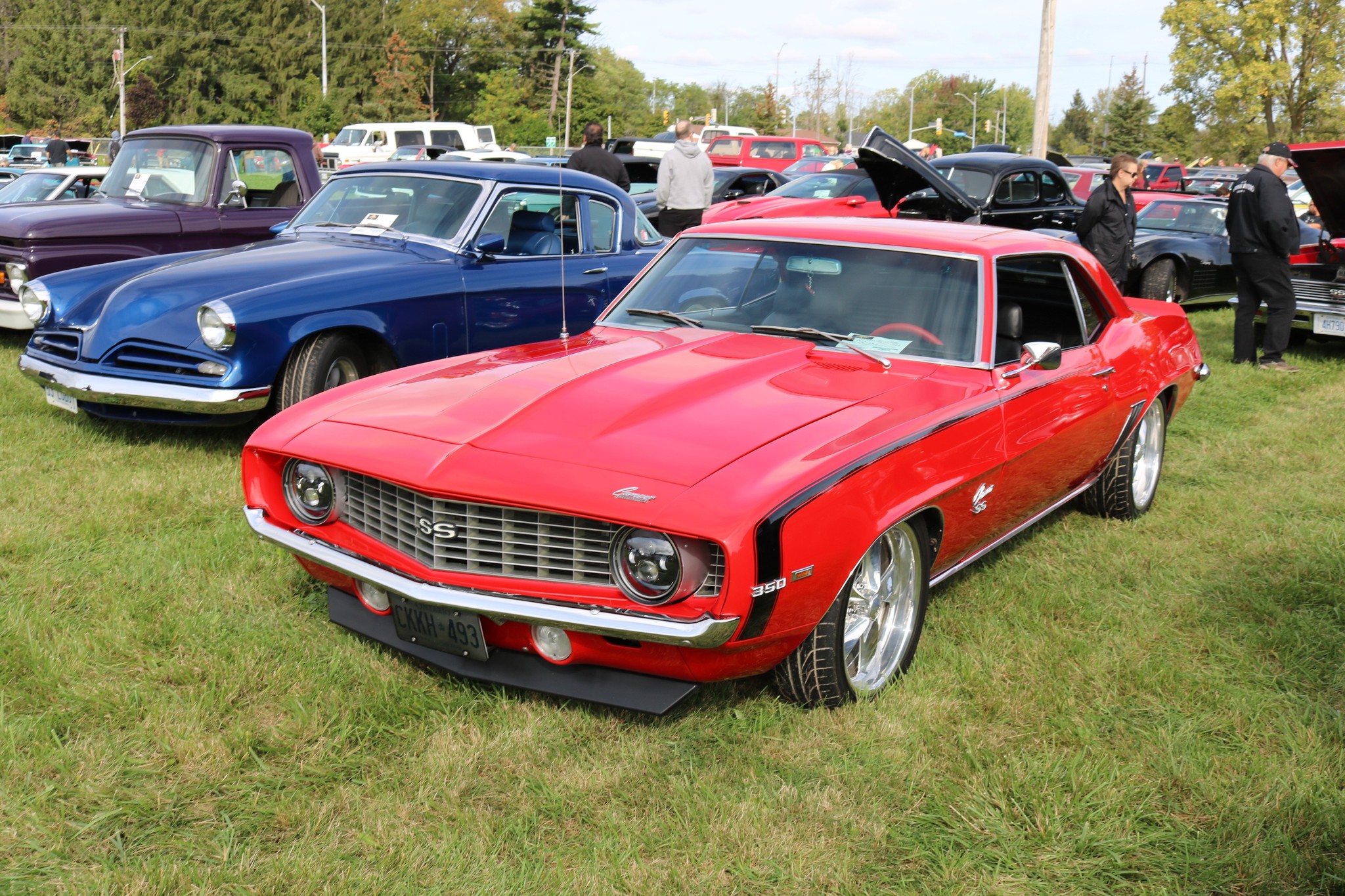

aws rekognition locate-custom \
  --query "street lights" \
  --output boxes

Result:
[953,93,977,149]
[776,42,786,130]
[908,76,927,140]
[564,65,595,148]
[121,56,152,144]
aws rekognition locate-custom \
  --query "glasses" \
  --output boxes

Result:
[1123,170,1137,178]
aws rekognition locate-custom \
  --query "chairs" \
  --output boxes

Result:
[755,277,819,332]
[403,197,452,238]
[496,208,563,258]
[264,181,300,206]
[959,301,1023,362]
[737,181,763,195]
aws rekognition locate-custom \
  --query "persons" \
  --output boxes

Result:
[0,159,10,168]
[308,131,324,167]
[561,121,631,224]
[656,120,715,239]
[231,149,295,183]
[1141,154,1327,234]
[506,142,516,152]
[1077,154,1138,296]
[22,130,73,167]
[1225,141,1303,372]
[108,131,168,168]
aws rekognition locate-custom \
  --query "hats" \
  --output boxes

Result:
[1239,161,1244,164]
[1262,142,1299,168]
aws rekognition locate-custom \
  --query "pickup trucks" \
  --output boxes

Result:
[0,125,455,329]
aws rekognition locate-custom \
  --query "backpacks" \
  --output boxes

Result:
[110,139,121,161]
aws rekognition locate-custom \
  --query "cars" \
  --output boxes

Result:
[18,160,778,429]
[353,123,1345,338]
[241,216,1215,717]
[1,134,238,206]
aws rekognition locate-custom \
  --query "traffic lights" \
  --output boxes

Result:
[705,114,710,127]
[663,110,668,125]
[984,120,992,133]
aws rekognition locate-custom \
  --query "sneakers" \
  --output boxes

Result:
[1260,361,1300,373]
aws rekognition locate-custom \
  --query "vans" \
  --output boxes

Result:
[317,122,495,171]
